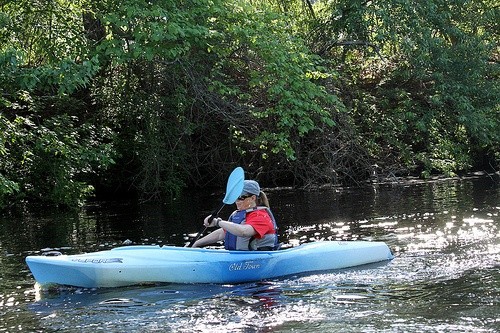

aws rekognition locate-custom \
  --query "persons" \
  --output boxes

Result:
[183,179,278,251]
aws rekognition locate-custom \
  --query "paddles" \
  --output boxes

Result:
[188,166,245,247]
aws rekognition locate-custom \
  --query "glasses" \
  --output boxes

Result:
[237,193,255,201]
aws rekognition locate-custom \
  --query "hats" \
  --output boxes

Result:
[241,179,260,196]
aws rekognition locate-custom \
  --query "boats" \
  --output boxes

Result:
[24,242,392,289]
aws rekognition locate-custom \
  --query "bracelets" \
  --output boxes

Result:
[216,217,222,228]
